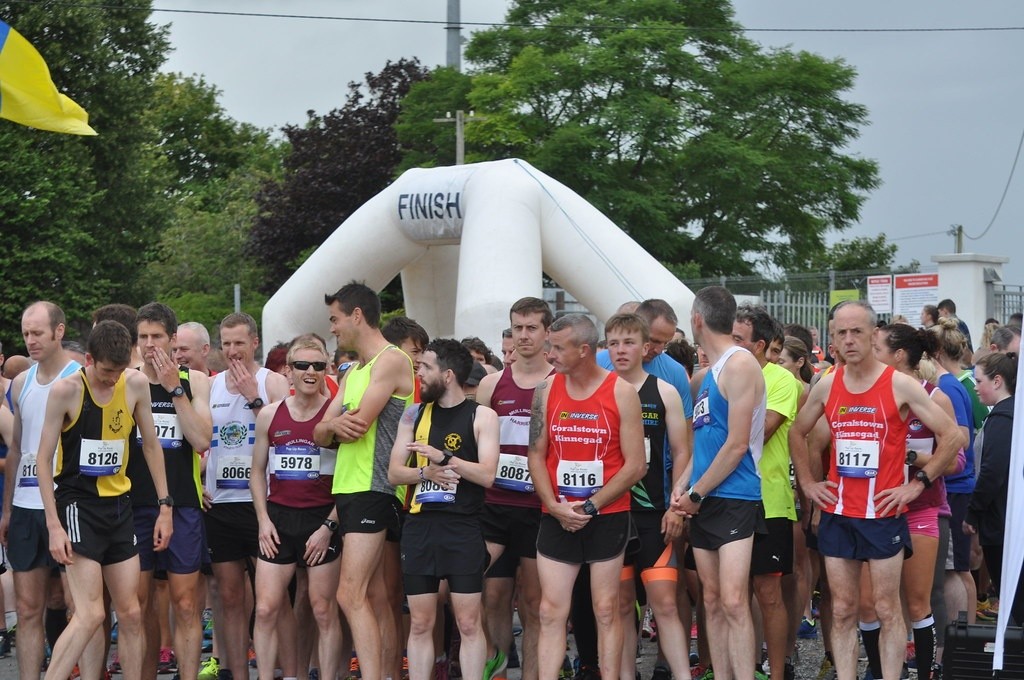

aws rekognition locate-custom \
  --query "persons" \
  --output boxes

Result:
[0,279,1024,680]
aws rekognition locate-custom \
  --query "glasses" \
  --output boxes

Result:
[290,361,327,371]
[337,361,359,372]
[648,342,672,351]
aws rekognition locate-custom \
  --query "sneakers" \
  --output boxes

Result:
[1,607,1023,680]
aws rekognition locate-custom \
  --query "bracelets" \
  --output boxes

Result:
[419,465,426,483]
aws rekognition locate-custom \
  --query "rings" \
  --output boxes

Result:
[317,553,321,557]
[159,364,163,367]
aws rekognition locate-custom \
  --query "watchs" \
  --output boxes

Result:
[437,449,453,466]
[905,450,916,465]
[158,495,173,506]
[913,470,931,489]
[688,486,705,503]
[322,519,338,533]
[169,385,185,398]
[249,398,264,409]
[581,499,598,517]
